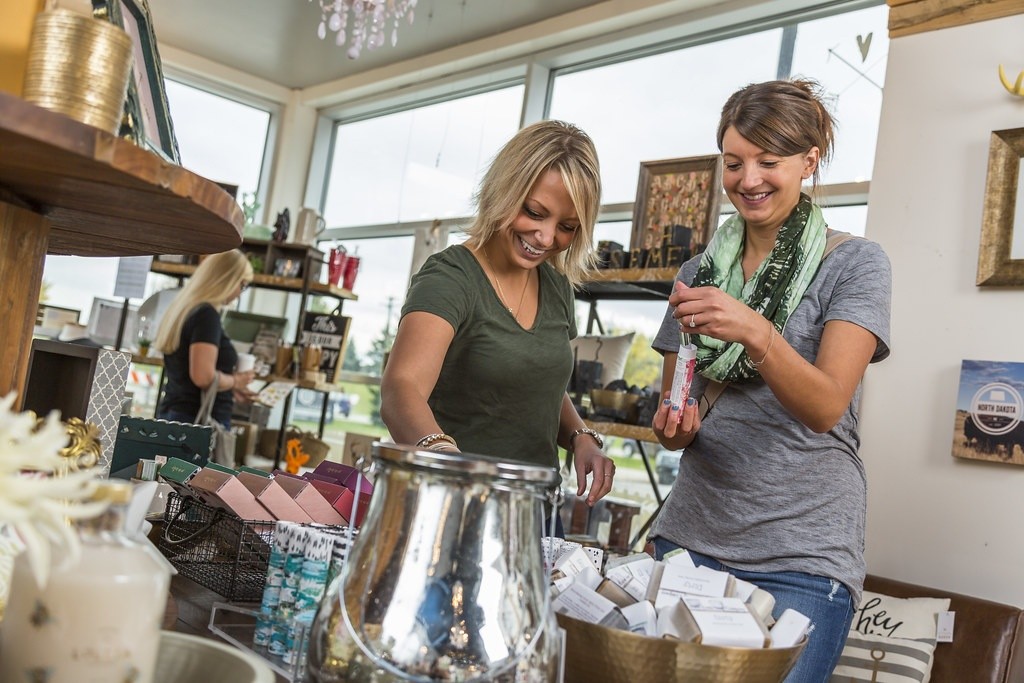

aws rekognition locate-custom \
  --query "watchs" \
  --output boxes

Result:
[569,428,604,452]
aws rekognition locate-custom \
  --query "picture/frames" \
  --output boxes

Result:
[94,0,179,164]
[632,154,725,272]
[975,126,1023,288]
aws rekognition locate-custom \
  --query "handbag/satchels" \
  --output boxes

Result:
[195,370,236,469]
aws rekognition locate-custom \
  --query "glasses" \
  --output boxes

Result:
[239,281,248,292]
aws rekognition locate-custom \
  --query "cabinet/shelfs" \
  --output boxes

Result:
[150,256,361,445]
[384,248,738,443]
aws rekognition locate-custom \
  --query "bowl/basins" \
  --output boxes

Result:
[590,389,640,420]
[153,631,275,683]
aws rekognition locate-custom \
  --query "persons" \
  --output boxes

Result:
[642,80,892,683]
[414,434,457,451]
[377,122,616,540]
[153,248,262,461]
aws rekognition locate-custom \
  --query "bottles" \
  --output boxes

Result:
[0,479,173,683]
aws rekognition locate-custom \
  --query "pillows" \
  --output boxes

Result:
[832,590,951,683]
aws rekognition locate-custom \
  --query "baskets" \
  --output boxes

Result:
[159,490,277,602]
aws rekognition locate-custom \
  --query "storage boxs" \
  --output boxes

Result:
[157,458,375,599]
[19,339,132,479]
[554,547,814,651]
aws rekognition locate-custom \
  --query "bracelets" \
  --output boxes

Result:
[748,320,774,367]
[232,376,236,389]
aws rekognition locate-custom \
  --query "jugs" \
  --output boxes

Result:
[293,208,326,245]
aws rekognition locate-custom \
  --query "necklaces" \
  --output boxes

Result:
[481,243,531,320]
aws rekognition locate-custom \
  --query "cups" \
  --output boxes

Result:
[325,248,348,287]
[276,346,293,377]
[341,257,359,292]
[237,353,257,373]
[302,343,323,371]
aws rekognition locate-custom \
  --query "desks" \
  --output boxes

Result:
[0,98,247,412]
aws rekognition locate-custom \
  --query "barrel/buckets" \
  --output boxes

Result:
[306,441,570,682]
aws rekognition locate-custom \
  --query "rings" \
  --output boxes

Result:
[604,473,612,477]
[690,314,696,328]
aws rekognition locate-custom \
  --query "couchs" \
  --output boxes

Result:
[832,573,1024,683]
